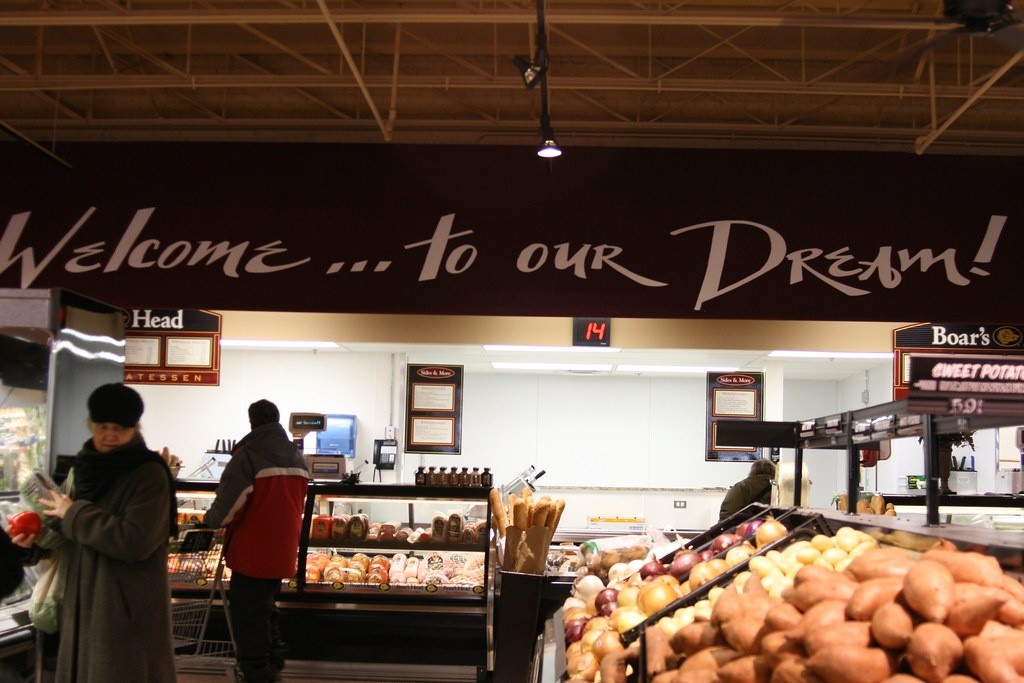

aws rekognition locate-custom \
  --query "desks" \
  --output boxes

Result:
[493,502,1024,683]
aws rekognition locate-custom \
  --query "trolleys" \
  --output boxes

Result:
[163,526,246,683]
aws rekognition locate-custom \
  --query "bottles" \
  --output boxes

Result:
[447,467,459,487]
[481,468,493,488]
[470,467,481,488]
[415,466,426,485]
[459,467,470,487]
[437,467,448,486]
[426,467,437,486]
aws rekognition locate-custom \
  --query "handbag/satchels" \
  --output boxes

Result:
[28,548,64,635]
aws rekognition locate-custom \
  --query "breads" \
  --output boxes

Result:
[365,513,488,547]
[306,552,391,583]
[488,486,566,540]
[838,492,895,515]
[160,447,181,467]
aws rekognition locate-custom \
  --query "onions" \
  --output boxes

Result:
[564,516,788,657]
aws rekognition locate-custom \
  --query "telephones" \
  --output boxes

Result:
[372,439,397,470]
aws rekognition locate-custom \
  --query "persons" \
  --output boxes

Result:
[202,399,310,683]
[720,459,776,521]
[5,382,177,683]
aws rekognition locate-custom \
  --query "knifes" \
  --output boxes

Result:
[950,455,975,472]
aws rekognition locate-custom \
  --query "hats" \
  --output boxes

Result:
[87,382,144,426]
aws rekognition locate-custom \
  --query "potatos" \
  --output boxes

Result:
[563,527,1024,683]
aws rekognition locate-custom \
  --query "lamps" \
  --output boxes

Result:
[511,55,544,86]
[537,115,563,158]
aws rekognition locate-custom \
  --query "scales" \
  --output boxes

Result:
[289,412,347,480]
